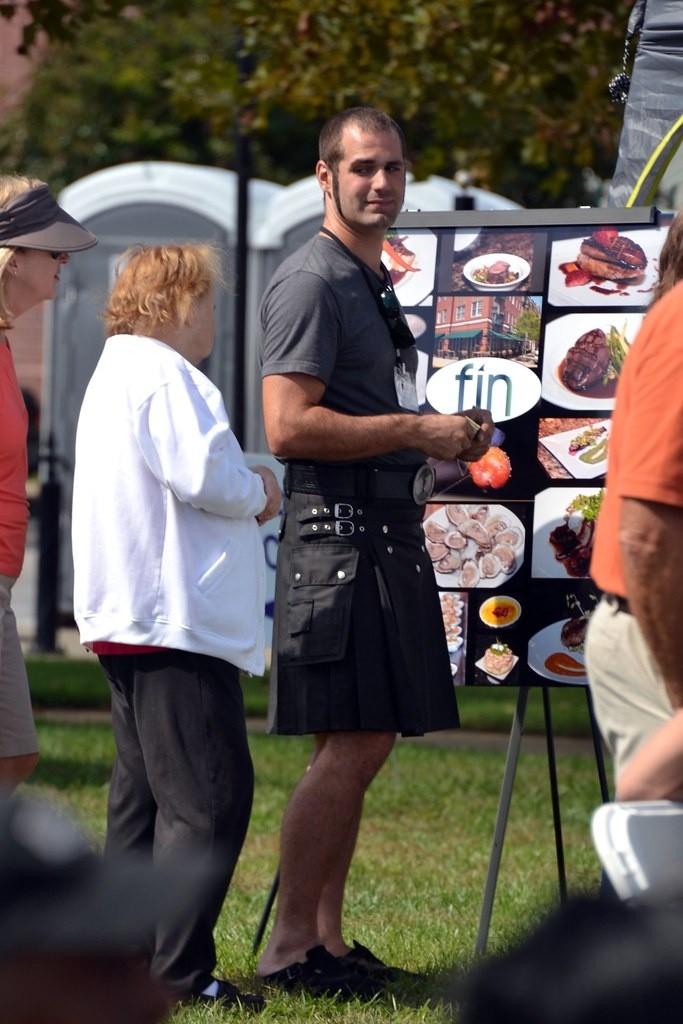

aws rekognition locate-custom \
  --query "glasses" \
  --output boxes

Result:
[18,246,59,260]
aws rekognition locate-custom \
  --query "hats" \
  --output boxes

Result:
[0,184,98,252]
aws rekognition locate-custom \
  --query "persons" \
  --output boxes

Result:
[0,175,99,803]
[457,894,683,1024]
[583,209,683,801]
[0,791,220,1024]
[71,243,283,1019]
[254,107,495,1004]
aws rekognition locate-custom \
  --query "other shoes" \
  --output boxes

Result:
[174,977,264,1014]
[263,943,386,1001]
[340,940,426,995]
